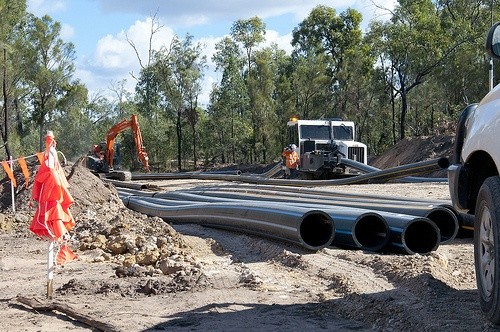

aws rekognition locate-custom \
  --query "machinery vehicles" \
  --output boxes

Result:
[286,117,368,180]
[78,113,151,182]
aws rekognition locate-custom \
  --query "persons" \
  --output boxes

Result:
[282,144,300,179]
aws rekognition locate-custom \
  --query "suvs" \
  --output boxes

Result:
[445,20,500,326]
